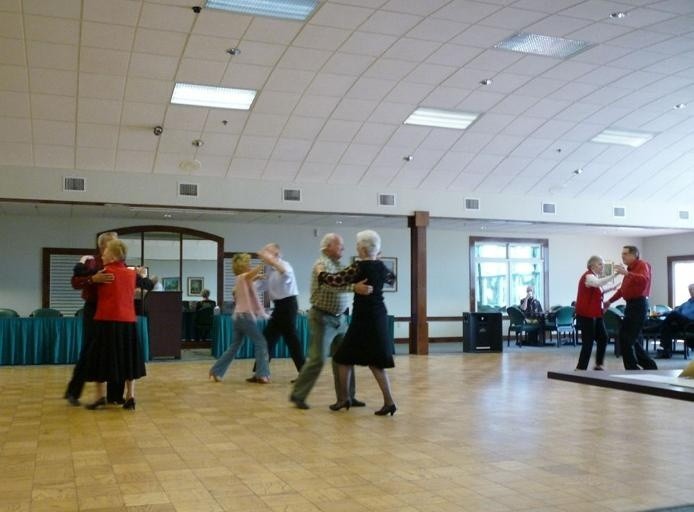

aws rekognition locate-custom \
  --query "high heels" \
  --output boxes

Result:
[329,399,351,411]
[374,404,396,416]
[208,370,223,381]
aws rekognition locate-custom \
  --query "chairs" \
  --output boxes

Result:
[76,307,86,315]
[266,308,274,315]
[0,307,18,317]
[29,308,62,316]
[477,302,694,359]
[194,300,216,332]
[222,300,235,315]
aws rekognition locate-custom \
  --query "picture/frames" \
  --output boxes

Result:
[187,277,204,296]
[162,277,180,291]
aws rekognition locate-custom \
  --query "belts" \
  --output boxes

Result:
[312,306,341,318]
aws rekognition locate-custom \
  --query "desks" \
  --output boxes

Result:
[1,315,150,365]
[546,369,694,403]
[182,311,216,342]
[210,311,396,360]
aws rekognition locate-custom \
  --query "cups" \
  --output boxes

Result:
[650,304,656,316]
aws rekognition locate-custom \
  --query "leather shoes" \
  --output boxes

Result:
[290,394,309,409]
[247,375,272,383]
[352,397,365,407]
[68,395,136,412]
[654,353,671,359]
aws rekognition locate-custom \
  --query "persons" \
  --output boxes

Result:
[602,245,657,371]
[245,243,306,383]
[573,255,623,371]
[63,231,165,411]
[207,253,272,384]
[223,287,238,315]
[657,283,694,358]
[289,233,373,409]
[315,229,397,417]
[520,287,546,343]
[200,289,209,301]
